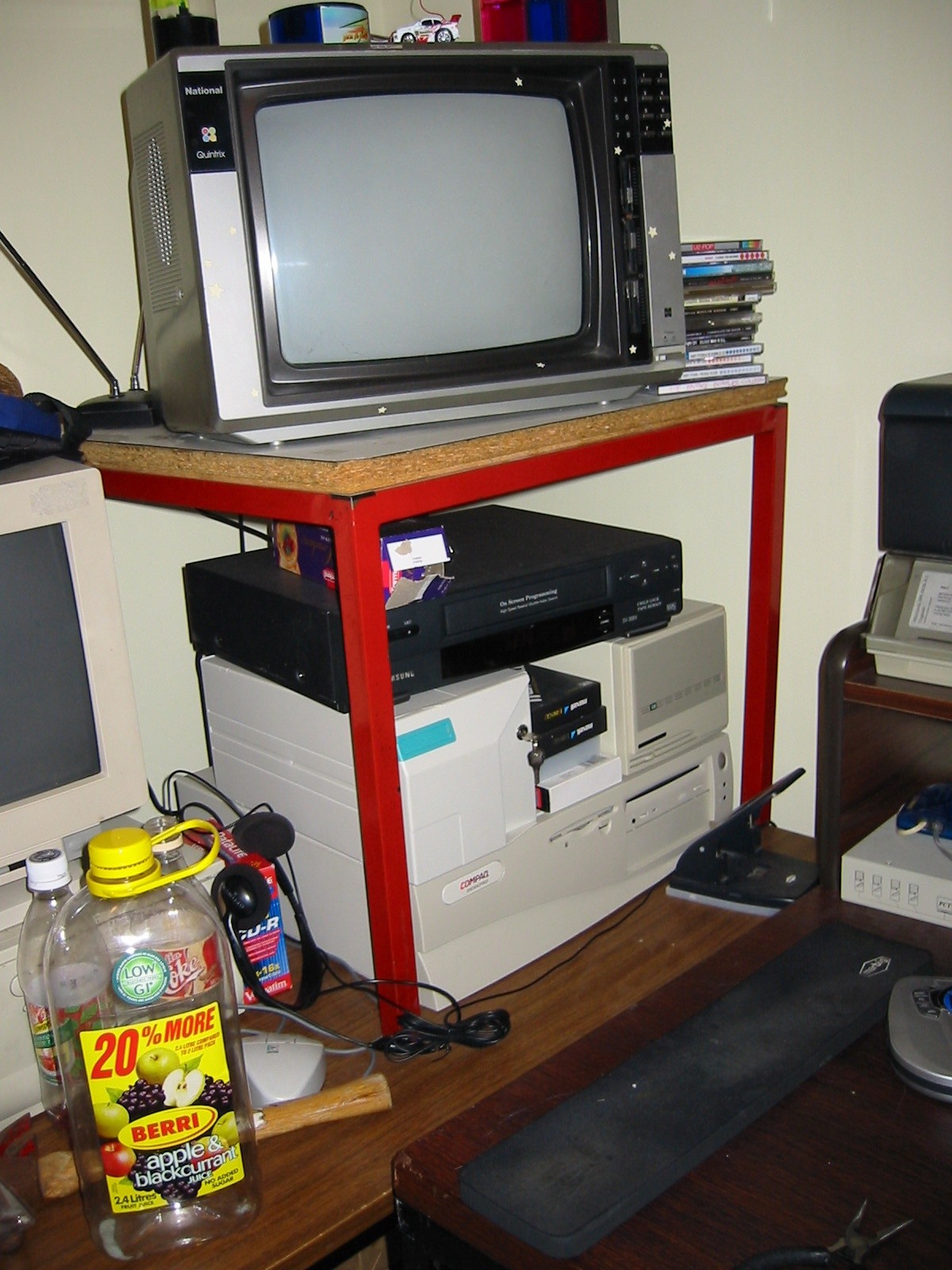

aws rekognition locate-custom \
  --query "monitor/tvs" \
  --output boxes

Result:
[0,453,148,889]
[120,45,690,450]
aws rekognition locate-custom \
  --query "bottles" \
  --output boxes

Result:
[43,818,263,1260]
[137,815,234,1089]
[16,851,121,1126]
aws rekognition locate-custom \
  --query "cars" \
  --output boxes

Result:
[389,14,462,44]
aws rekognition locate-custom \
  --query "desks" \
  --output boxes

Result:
[390,619,952,1270]
[80,375,790,1039]
[0,823,807,1270]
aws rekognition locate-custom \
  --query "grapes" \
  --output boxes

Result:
[116,1076,233,1205]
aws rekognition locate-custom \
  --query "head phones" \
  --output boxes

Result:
[210,811,321,1012]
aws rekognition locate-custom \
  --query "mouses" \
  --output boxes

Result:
[241,1031,327,1105]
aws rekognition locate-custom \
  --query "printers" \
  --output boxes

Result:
[856,369,952,689]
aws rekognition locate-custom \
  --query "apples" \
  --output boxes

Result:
[191,1111,240,1173]
[92,1101,136,1178]
[136,1047,206,1108]
